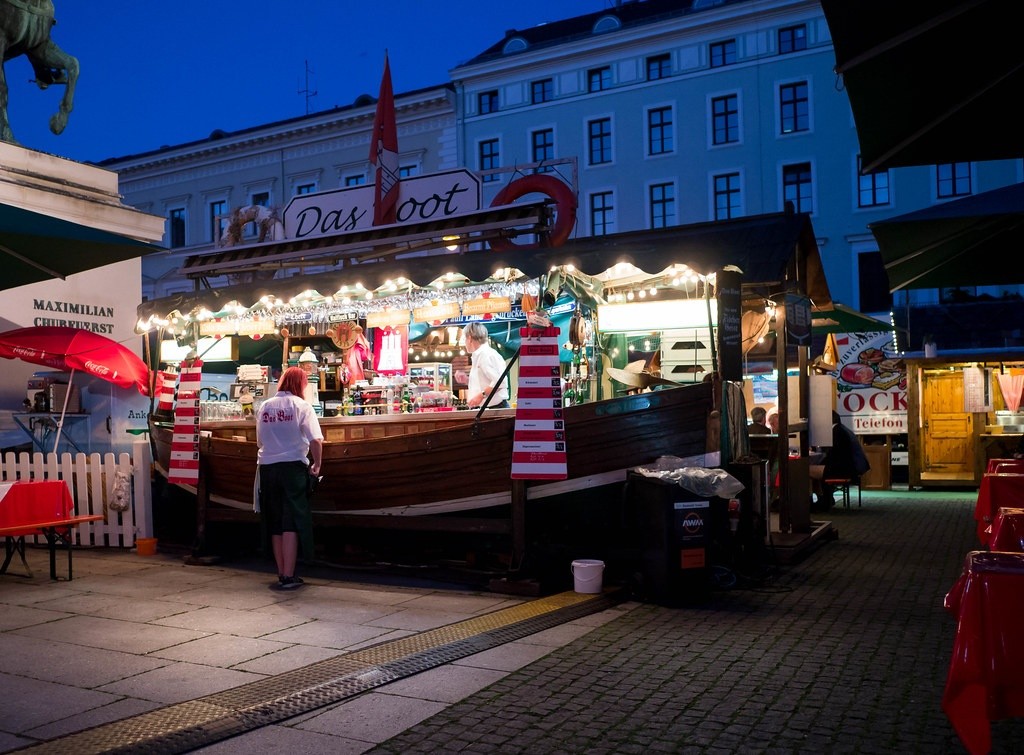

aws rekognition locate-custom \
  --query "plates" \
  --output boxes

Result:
[50,384,80,412]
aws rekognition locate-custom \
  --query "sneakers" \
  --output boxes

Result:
[278,574,304,587]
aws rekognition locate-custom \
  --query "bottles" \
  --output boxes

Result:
[344,372,476,416]
[200,401,245,420]
[561,347,589,408]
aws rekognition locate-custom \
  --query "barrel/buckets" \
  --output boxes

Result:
[136,538,157,555]
[571,559,605,593]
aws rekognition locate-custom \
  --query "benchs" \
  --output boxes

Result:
[824,476,862,509]
[0,515,105,581]
[946,456,1024,755]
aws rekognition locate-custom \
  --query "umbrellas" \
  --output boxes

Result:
[869,184,1024,295]
[821,0,1024,175]
[0,326,164,454]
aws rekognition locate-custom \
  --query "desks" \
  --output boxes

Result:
[0,478,74,577]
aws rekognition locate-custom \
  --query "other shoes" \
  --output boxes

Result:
[818,497,835,506]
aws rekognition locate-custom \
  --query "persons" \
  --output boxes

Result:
[462,323,510,410]
[257,366,324,588]
[748,407,871,512]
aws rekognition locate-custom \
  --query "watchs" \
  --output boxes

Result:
[482,391,486,398]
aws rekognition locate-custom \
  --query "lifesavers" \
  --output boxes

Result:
[481,171,577,251]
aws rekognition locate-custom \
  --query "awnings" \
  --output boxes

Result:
[768,302,895,338]
